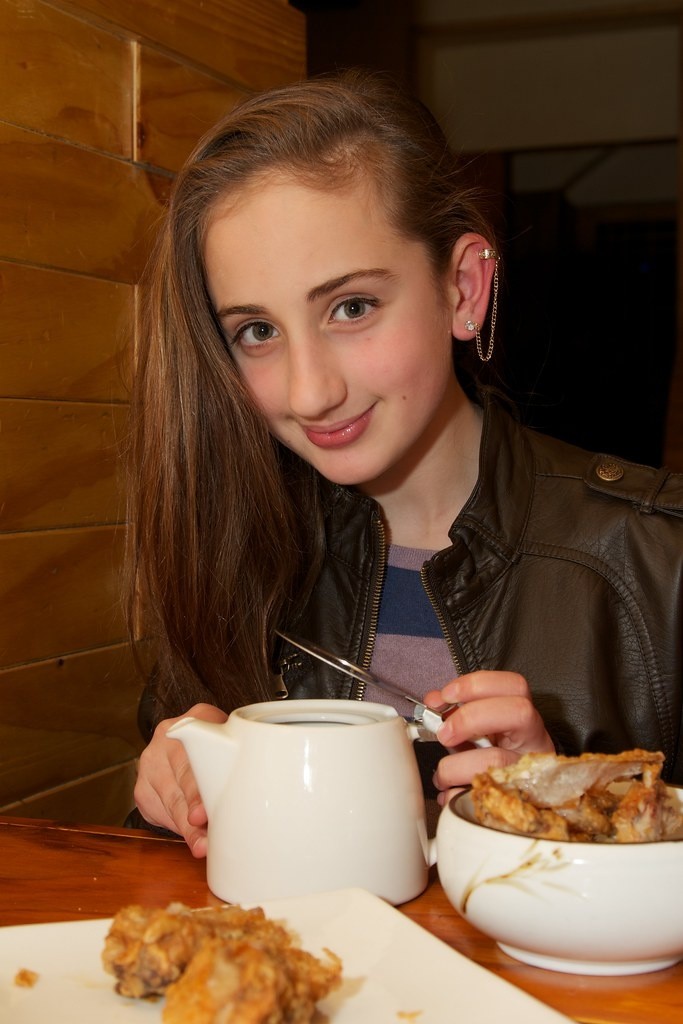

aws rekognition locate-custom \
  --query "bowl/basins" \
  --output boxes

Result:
[437,786,683,975]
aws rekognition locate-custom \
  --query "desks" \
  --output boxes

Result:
[2,807,682,1022]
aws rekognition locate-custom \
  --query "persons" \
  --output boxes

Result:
[108,65,683,859]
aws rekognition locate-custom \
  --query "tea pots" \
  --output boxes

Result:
[163,631,494,907]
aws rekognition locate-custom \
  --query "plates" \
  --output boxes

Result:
[0,886,578,1024]
